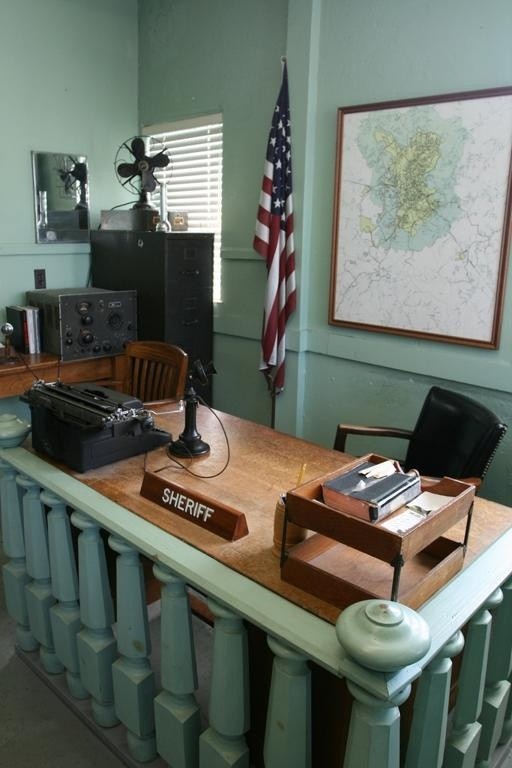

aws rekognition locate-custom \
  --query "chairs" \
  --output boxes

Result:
[97,340,189,407]
[333,387,508,496]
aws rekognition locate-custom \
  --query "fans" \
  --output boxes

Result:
[114,135,175,211]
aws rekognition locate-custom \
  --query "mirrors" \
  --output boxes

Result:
[31,151,91,245]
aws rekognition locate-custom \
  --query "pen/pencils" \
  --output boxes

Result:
[297,462,306,486]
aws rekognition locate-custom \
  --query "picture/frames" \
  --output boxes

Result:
[328,86,512,350]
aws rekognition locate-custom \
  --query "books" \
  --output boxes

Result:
[319,460,422,524]
[5,304,44,354]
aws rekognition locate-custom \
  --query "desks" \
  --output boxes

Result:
[18,396,511,624]
[0,342,134,398]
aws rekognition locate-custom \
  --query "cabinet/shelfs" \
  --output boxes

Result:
[89,229,215,409]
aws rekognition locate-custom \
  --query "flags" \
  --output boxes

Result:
[250,55,295,391]
